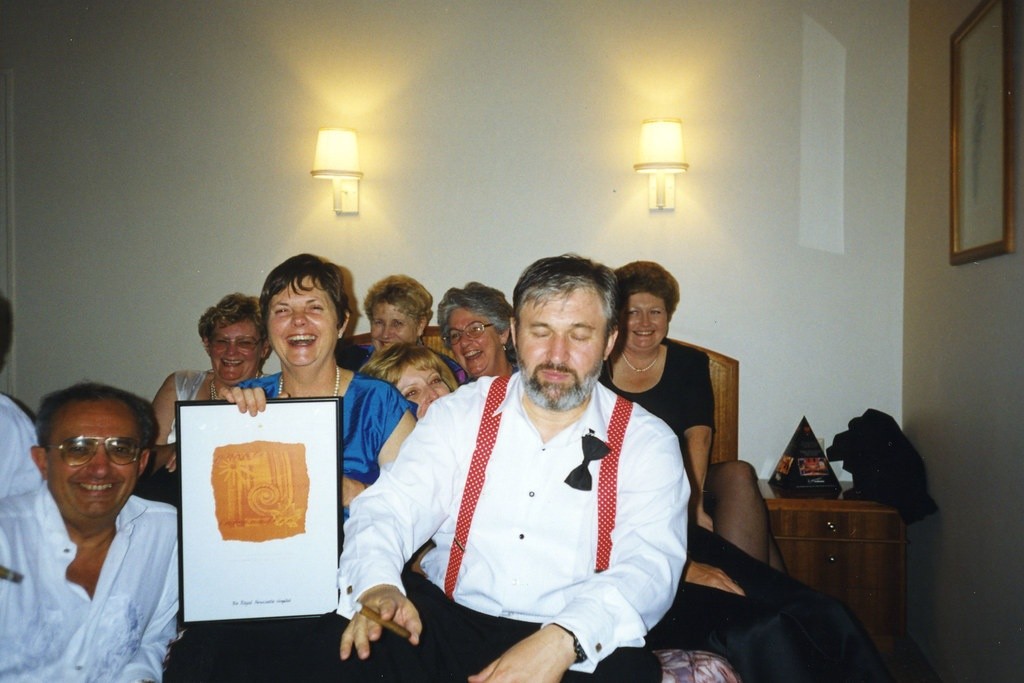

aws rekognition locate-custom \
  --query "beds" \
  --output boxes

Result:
[352,327,740,683]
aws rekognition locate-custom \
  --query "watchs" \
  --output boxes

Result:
[551,623,587,664]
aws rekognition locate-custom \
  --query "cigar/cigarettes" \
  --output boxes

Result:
[353,601,412,641]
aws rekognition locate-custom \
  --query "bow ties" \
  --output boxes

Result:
[563,431,611,491]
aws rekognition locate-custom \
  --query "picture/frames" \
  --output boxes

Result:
[174,396,344,628]
[949,0,1016,266]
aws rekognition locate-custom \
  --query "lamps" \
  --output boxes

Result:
[632,116,688,213]
[309,125,364,214]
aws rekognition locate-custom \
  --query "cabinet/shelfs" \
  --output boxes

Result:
[757,479,909,654]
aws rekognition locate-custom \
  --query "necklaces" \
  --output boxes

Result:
[622,345,660,372]
[210,371,264,400]
[278,365,340,397]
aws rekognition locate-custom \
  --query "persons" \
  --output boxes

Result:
[0,293,40,498]
[359,274,519,382]
[0,382,178,683]
[355,342,896,682]
[216,253,419,523]
[598,261,787,574]
[334,254,692,683]
[152,292,273,473]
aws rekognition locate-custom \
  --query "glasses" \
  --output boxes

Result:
[447,319,495,345]
[44,434,151,466]
[207,330,265,353]
